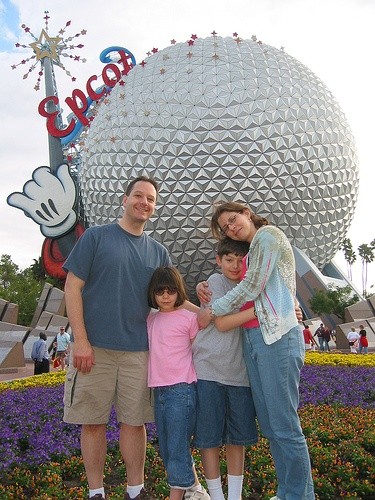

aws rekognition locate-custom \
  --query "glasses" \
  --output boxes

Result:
[219,210,240,236]
[154,288,178,296]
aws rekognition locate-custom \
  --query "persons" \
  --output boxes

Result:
[196,202,315,500]
[31,326,71,375]
[303,323,368,354]
[146,266,210,500]
[191,237,303,500]
[62,177,201,500]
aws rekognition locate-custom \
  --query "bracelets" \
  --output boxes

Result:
[254,310,257,317]
[209,305,215,321]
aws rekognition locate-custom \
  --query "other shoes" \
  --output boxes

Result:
[184,486,210,500]
[123,488,159,500]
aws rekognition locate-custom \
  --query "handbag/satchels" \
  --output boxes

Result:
[349,342,354,346]
